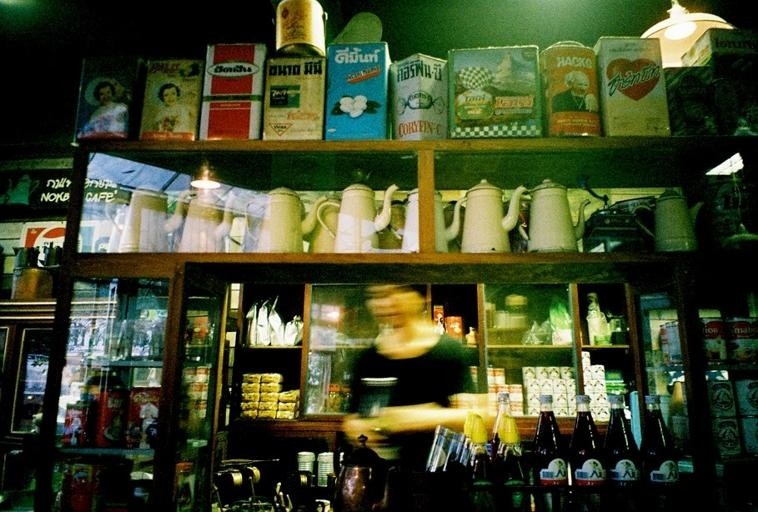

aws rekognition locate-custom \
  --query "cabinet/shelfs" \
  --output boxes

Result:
[226,280,644,472]
[34,137,758,512]
[0,294,212,510]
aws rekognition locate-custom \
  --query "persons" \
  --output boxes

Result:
[341,266,481,468]
[89,81,127,131]
[153,82,196,135]
[552,69,598,113]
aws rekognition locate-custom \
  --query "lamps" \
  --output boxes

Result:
[641,0,732,65]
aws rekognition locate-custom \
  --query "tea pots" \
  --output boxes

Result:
[390,185,468,252]
[307,194,340,256]
[332,434,399,509]
[376,194,410,250]
[106,185,190,252]
[512,178,591,252]
[445,179,528,254]
[243,186,327,252]
[175,189,238,252]
[316,183,398,254]
[632,186,705,251]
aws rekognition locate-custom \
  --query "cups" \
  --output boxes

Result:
[425,423,466,474]
[318,452,336,487]
[297,451,314,473]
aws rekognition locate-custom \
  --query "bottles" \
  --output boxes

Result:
[604,394,640,483]
[665,321,682,359]
[491,392,512,452]
[570,395,606,487]
[668,381,686,439]
[463,411,491,481]
[700,317,727,362]
[640,395,680,483]
[532,395,568,487]
[706,380,736,418]
[713,417,741,459]
[169,462,195,512]
[741,416,758,453]
[495,412,526,482]
[735,380,758,417]
[658,324,669,354]
[727,318,758,365]
